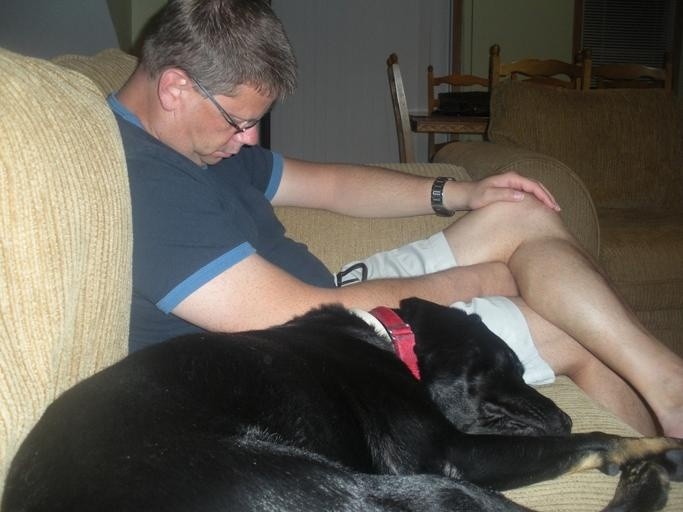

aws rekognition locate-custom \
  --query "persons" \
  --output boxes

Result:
[101,1,682,438]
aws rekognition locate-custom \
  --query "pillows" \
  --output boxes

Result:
[489,77,682,215]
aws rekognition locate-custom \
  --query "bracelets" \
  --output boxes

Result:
[431,175,458,217]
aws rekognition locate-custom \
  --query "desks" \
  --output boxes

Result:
[409,115,490,134]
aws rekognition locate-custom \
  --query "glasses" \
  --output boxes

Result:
[190,77,257,133]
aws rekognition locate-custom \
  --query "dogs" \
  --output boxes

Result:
[0,295,681,511]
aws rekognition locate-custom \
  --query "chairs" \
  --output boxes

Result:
[489,44,591,94]
[428,64,488,162]
[575,51,672,92]
[387,51,418,163]
[431,77,683,359]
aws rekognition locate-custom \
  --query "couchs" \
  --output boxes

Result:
[1,47,648,511]
